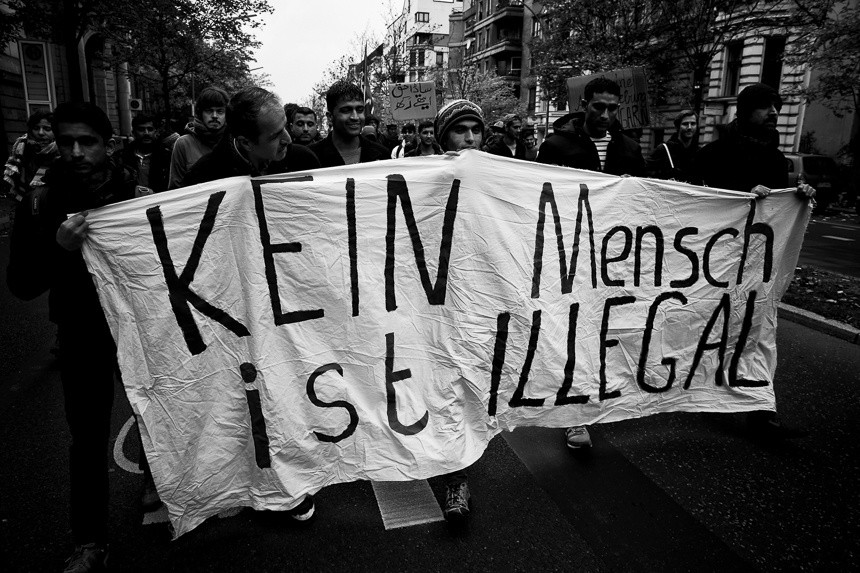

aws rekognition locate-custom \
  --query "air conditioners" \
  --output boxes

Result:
[129,99,142,111]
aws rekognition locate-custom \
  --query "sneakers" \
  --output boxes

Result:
[142,470,161,511]
[444,479,470,518]
[566,424,593,449]
[61,544,107,573]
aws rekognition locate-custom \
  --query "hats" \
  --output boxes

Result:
[737,84,781,118]
[362,126,378,142]
[434,99,485,146]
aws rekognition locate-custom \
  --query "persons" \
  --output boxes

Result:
[691,84,816,430]
[4,74,707,573]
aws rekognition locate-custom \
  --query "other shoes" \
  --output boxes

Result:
[292,496,316,521]
